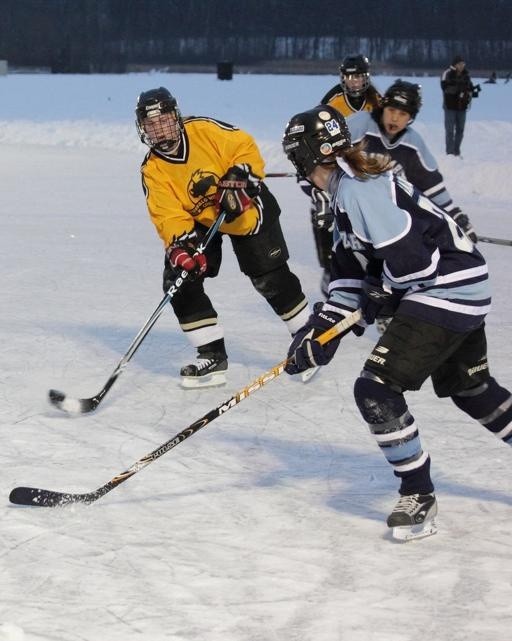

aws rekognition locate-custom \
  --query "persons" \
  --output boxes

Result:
[312,55,385,266]
[314,78,475,296]
[136,89,323,389]
[283,105,512,542]
[442,57,479,156]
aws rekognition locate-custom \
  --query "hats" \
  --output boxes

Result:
[452,54,463,65]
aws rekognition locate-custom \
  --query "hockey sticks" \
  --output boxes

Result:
[9,308,364,505]
[50,209,226,414]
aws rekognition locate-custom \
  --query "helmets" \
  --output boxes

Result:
[378,79,422,119]
[340,54,371,97]
[135,87,182,150]
[281,104,351,183]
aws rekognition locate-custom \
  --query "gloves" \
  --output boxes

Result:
[362,272,388,325]
[285,310,350,376]
[215,168,253,223]
[166,242,207,281]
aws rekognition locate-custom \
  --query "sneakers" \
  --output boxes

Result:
[181,353,227,377]
[387,494,438,527]
